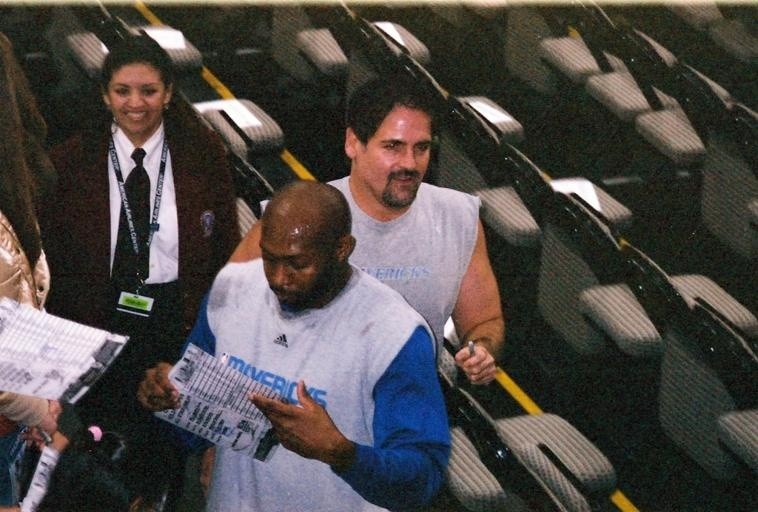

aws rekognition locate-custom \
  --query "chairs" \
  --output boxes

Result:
[25,1,758,512]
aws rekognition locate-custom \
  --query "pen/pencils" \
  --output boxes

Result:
[40,430,52,443]
[468,341,489,387]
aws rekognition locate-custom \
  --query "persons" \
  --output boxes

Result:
[200,75,505,501]
[136,180,452,512]
[40,43,241,512]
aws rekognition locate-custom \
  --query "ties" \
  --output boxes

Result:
[112,148,150,291]
